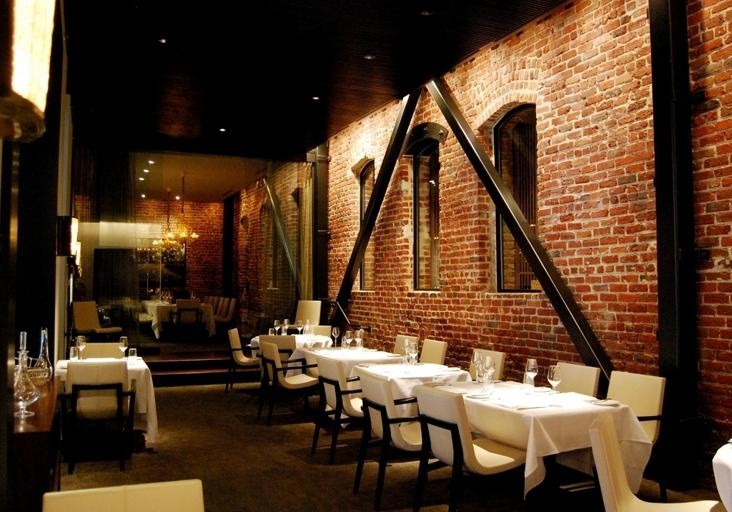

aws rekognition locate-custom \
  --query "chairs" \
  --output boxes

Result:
[42,478,206,512]
[57,363,136,474]
[73,342,127,358]
[72,301,123,335]
[119,294,236,341]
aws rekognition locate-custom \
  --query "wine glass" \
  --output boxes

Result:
[346,331,353,352]
[296,320,303,335]
[355,330,363,353]
[547,365,564,405]
[525,359,538,392]
[484,355,495,391]
[409,343,419,369]
[274,320,281,335]
[332,327,340,350]
[147,288,173,305]
[119,336,128,360]
[70,347,78,361]
[402,339,410,366]
[305,320,317,337]
[284,319,290,335]
[191,292,196,300]
[367,348,377,352]
[77,335,86,360]
[471,350,482,382]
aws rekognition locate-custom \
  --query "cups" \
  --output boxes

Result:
[341,336,347,350]
[129,348,137,365]
[27,368,45,385]
[269,329,273,335]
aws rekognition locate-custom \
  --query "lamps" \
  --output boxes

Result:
[150,173,200,261]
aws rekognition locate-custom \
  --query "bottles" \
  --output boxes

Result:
[35,327,53,382]
[136,246,186,263]
[15,332,40,417]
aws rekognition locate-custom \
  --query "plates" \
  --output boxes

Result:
[442,367,460,371]
[466,393,490,399]
[386,353,401,356]
[360,362,376,367]
[501,381,518,386]
[534,387,549,391]
[592,400,619,406]
[133,356,142,360]
[435,385,468,393]
